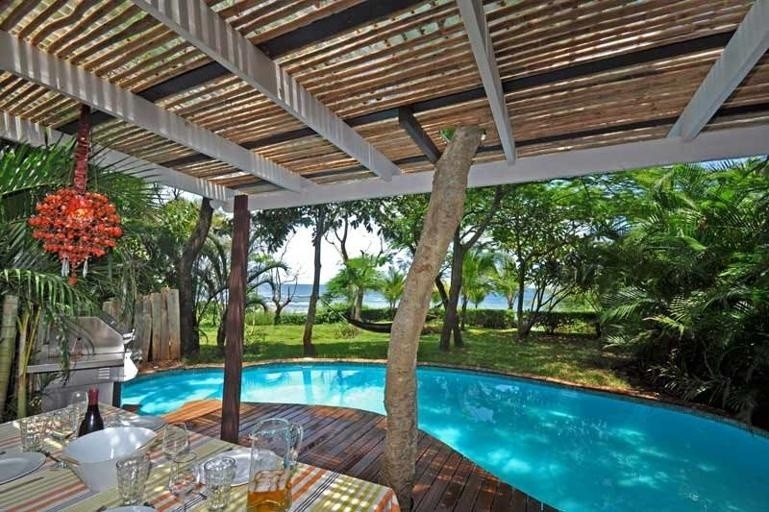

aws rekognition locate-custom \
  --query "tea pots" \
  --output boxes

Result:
[246,417,303,512]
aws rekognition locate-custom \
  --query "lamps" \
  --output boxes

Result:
[28,103,123,282]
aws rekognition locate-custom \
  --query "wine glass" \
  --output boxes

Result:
[162,421,200,512]
[51,409,78,470]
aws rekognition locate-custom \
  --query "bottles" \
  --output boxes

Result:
[78,388,103,437]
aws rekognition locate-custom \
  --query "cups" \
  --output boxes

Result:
[204,456,237,511]
[116,453,151,506]
[19,416,44,452]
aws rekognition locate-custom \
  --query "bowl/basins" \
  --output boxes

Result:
[62,427,158,492]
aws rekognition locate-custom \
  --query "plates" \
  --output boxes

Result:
[1,451,46,485]
[114,415,167,431]
[199,447,250,486]
